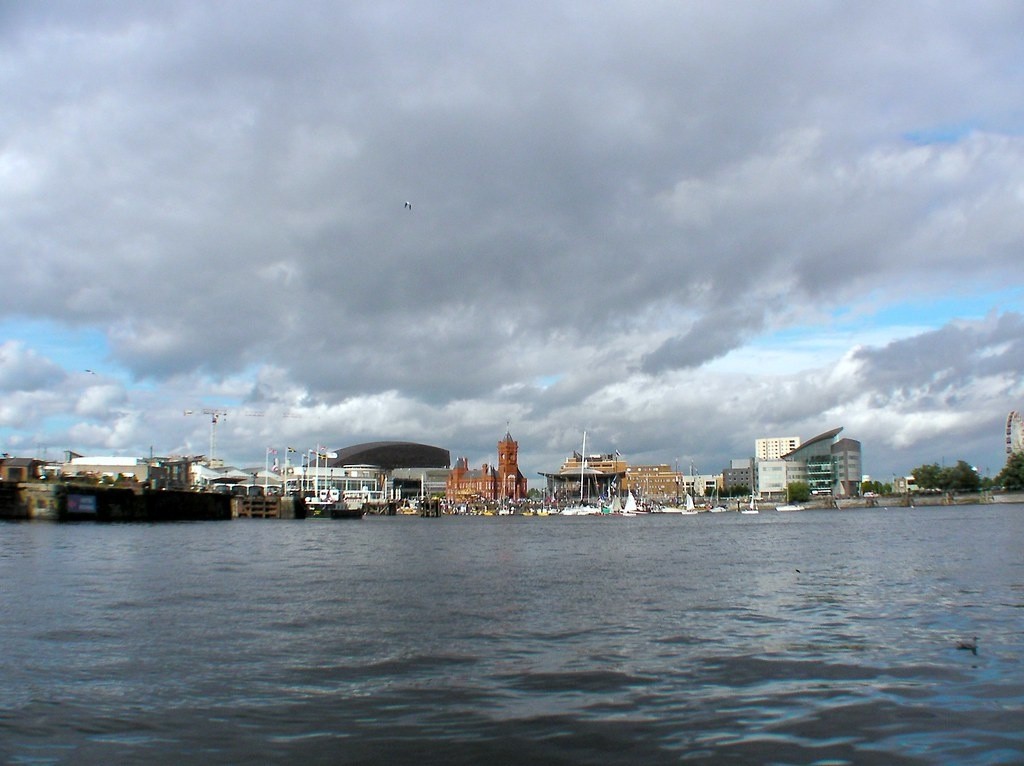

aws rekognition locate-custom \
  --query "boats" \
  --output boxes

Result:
[476,430,763,516]
[774,503,806,512]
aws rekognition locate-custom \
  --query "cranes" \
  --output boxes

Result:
[181,406,306,470]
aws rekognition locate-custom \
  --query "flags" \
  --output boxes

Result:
[610,476,617,493]
[267,445,337,471]
[616,450,621,457]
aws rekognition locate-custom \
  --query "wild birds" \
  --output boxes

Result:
[405,202,411,210]
[796,569,800,573]
[85,370,95,374]
[955,636,980,648]
